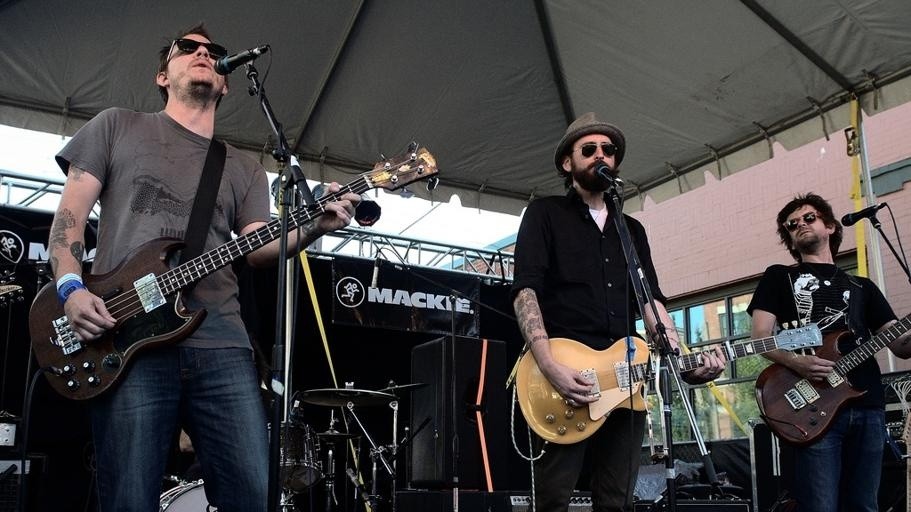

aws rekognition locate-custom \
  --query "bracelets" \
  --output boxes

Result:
[56,273,84,306]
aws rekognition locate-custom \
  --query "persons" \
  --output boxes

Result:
[509,111,728,512]
[745,193,911,512]
[49,22,363,512]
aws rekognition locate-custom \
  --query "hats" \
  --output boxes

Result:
[553,110,624,176]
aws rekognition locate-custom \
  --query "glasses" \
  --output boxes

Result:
[160,37,226,69]
[780,208,823,232]
[567,139,620,159]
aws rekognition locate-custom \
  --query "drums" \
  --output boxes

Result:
[280,419,320,491]
[158,478,211,512]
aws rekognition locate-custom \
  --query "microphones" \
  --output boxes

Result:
[594,162,624,188]
[842,203,884,226]
[214,44,269,74]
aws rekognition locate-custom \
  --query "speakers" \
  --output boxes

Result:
[411,336,507,488]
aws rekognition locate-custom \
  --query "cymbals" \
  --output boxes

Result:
[304,389,399,408]
[378,382,423,389]
[317,430,355,438]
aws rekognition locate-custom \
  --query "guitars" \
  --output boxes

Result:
[756,313,911,443]
[30,141,440,400]
[516,324,823,445]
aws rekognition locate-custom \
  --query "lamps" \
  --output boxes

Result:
[269,171,383,226]
